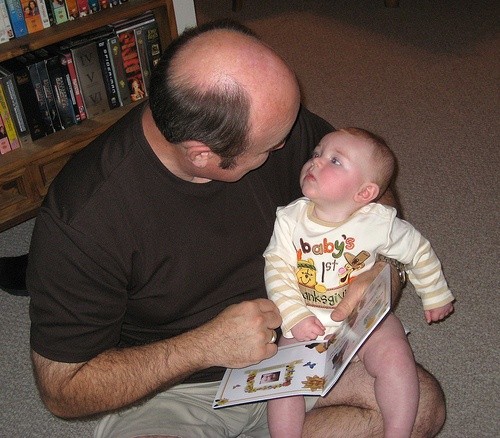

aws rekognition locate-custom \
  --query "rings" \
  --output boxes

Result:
[268,330,276,344]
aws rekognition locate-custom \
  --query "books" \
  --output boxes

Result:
[1,15,169,156]
[1,1,133,44]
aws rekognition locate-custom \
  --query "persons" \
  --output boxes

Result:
[28,19,447,437]
[262,127,457,438]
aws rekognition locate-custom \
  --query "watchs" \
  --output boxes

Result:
[376,253,407,286]
